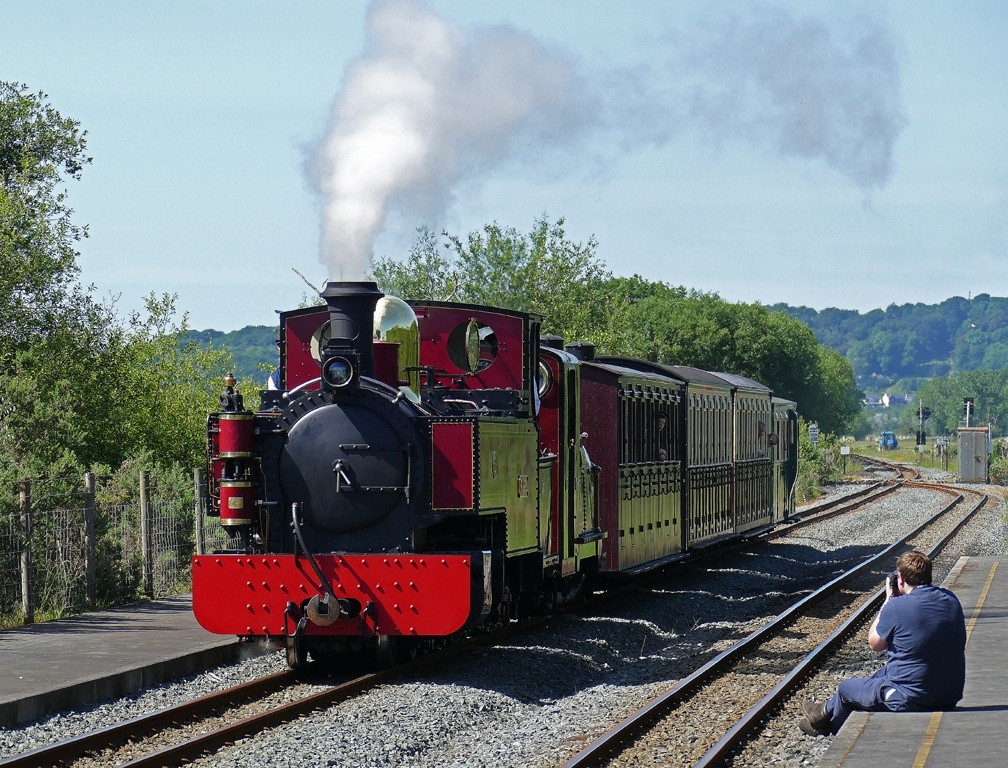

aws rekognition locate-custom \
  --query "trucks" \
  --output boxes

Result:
[879,431,899,448]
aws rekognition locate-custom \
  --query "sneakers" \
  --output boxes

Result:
[802,700,829,729]
[799,718,822,737]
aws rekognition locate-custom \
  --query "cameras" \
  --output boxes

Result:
[889,571,899,590]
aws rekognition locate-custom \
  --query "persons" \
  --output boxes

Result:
[657,411,669,463]
[758,421,778,458]
[800,551,967,737]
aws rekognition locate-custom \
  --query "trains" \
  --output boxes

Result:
[192,281,797,676]
